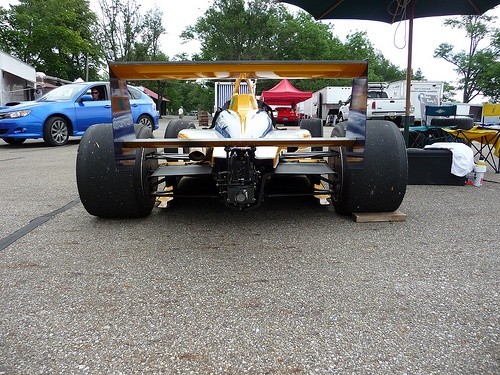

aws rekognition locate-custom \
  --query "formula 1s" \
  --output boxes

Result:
[76,61,408,218]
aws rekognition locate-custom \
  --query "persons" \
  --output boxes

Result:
[91,88,101,101]
[179,106,184,119]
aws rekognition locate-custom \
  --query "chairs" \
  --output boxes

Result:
[400,103,500,172]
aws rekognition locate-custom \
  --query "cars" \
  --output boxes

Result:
[273,107,299,125]
[0,82,159,146]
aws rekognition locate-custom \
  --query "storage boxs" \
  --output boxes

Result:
[408,148,466,185]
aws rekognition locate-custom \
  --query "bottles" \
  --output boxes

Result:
[474,153,487,186]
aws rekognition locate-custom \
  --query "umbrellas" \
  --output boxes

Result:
[280,0,500,147]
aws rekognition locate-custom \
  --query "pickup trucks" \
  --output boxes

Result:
[338,91,409,127]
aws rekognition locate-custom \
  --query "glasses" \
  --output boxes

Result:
[91,92,99,95]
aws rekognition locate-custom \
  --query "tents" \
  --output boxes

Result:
[258,79,313,120]
